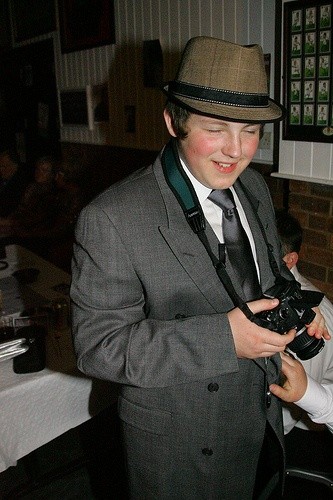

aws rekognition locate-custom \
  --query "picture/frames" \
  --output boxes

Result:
[8,0,60,49]
[57,0,116,55]
[282,0,333,144]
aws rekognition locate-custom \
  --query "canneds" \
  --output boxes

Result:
[52,298,69,329]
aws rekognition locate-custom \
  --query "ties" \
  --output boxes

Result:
[208,188,260,304]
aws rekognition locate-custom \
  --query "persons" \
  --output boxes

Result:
[307,35,314,52]
[292,106,299,122]
[322,7,329,25]
[320,82,327,96]
[293,12,299,30]
[292,37,300,53]
[292,83,299,101]
[269,209,333,470]
[305,83,313,99]
[306,59,314,76]
[321,58,328,76]
[0,149,35,218]
[0,160,64,238]
[306,11,314,28]
[320,33,329,50]
[42,164,87,270]
[69,35,331,500]
[319,107,325,122]
[292,60,299,74]
[305,107,312,121]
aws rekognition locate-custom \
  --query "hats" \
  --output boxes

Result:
[162,36,285,125]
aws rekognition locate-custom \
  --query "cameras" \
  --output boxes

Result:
[259,283,325,361]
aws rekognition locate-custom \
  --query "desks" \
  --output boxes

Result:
[0,244,123,475]
